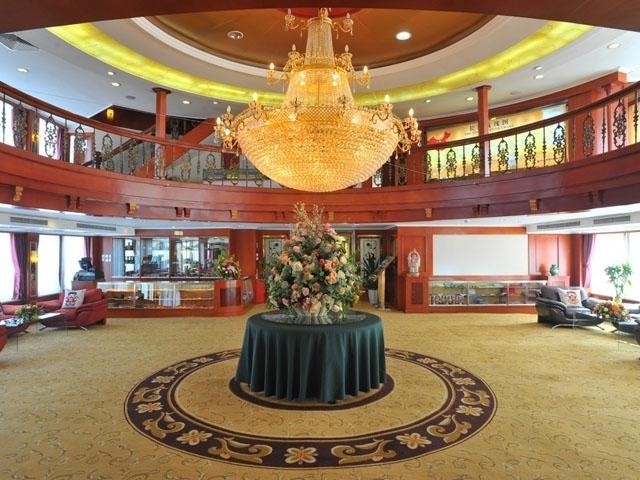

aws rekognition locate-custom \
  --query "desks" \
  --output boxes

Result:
[247,309,386,402]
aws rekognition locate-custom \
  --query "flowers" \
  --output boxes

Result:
[264,198,380,314]
[208,248,243,281]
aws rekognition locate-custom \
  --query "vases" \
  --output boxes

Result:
[549,264,559,276]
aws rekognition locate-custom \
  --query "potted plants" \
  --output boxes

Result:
[362,251,379,306]
[605,262,633,304]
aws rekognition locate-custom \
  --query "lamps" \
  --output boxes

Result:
[212,8,421,194]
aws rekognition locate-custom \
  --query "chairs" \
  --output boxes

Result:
[534,285,640,345]
[0,287,108,352]
